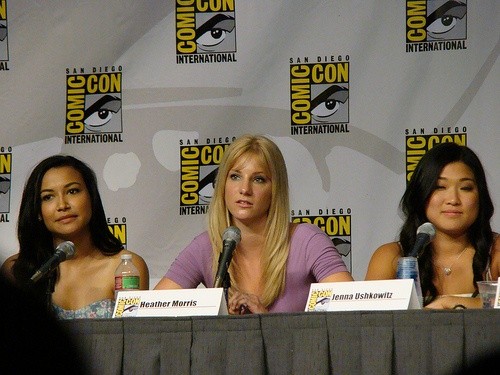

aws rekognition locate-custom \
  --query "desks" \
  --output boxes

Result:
[62,308,500,375]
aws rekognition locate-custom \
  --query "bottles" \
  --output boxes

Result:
[114,254,140,305]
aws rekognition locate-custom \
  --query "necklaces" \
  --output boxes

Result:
[444,248,465,275]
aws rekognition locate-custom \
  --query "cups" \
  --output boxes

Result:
[477,281,498,309]
[396,256,424,308]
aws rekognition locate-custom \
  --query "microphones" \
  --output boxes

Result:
[408,221,436,261]
[25,240,75,293]
[214,225,241,288]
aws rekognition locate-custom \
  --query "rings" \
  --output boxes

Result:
[236,292,240,296]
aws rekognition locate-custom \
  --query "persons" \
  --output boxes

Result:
[365,143,500,308]
[2,155,149,321]
[154,134,355,314]
